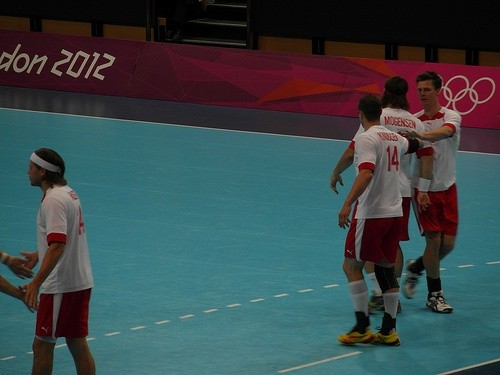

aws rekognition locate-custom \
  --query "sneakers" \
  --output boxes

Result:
[338,329,376,345]
[426,290,454,313]
[367,294,401,313]
[370,331,401,347]
[402,259,424,299]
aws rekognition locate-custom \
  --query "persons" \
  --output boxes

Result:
[0,250,40,313]
[401,70,461,314]
[330,75,435,314]
[338,92,424,346]
[19,148,95,375]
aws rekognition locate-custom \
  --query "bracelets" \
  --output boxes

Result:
[418,176,431,192]
[1,252,8,264]
